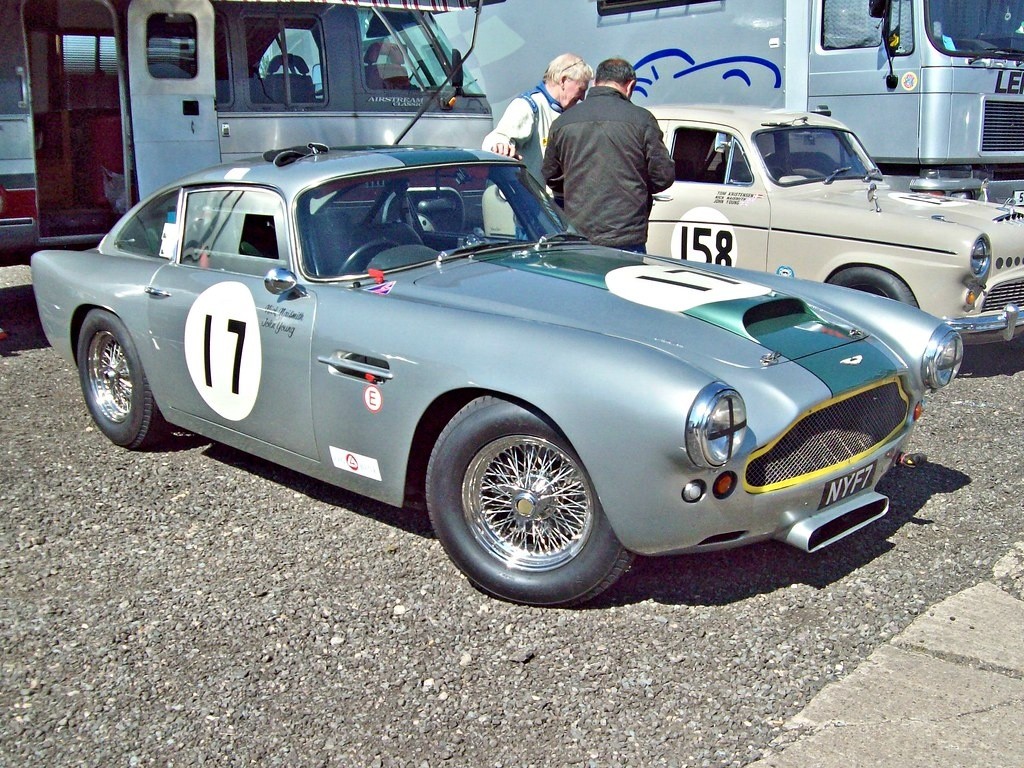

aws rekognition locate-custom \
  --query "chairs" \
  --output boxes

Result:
[262,54,315,102]
[305,212,358,277]
[363,42,411,89]
[365,223,425,270]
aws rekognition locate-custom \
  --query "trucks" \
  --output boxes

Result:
[273,1,1024,209]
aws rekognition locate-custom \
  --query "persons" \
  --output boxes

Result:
[481,53,595,241]
[540,59,676,256]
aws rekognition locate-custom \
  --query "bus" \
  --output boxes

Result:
[0,0,506,267]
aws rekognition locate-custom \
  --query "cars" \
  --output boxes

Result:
[29,145,964,610]
[482,105,1024,347]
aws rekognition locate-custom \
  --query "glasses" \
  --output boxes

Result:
[562,58,586,70]
